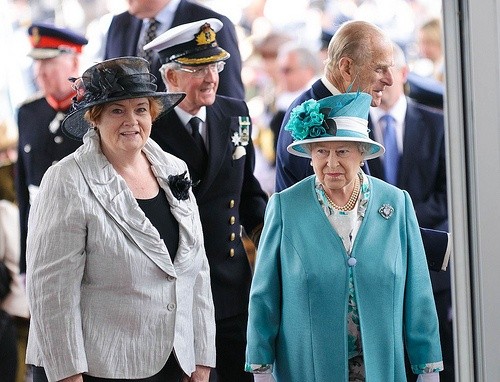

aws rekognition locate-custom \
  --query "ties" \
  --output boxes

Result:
[190,117,208,161]
[383,115,401,188]
[140,18,158,67]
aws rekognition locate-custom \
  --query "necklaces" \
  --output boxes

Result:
[319,172,360,212]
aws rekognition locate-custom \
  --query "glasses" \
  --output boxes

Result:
[179,61,227,79]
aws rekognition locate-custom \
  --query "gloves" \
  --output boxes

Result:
[254,373,275,382]
[416,373,440,382]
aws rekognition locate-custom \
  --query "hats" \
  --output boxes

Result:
[26,23,88,60]
[61,56,187,141]
[142,17,230,67]
[284,73,385,161]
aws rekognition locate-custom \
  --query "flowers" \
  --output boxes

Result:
[285,100,337,141]
[66,66,131,107]
[169,171,199,200]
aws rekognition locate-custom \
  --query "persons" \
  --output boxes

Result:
[243,28,295,197]
[0,163,35,382]
[1,0,444,110]
[24,56,218,382]
[354,38,457,382]
[253,45,321,169]
[14,19,95,281]
[244,93,445,382]
[274,20,450,275]
[141,16,271,382]
